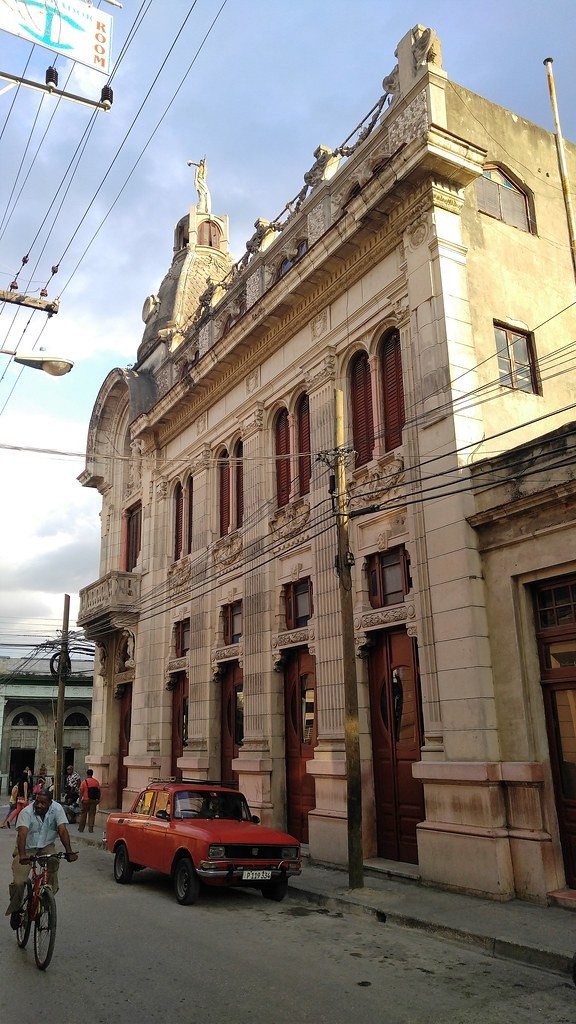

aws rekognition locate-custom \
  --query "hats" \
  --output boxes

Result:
[38,789,52,795]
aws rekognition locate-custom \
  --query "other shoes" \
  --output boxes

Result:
[10,911,21,930]
[7,820,11,829]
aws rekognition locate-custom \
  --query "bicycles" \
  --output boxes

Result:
[16,849,79,970]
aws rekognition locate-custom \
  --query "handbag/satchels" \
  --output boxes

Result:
[17,797,25,804]
[88,786,101,800]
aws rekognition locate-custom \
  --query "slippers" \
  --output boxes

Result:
[0,825,6,829]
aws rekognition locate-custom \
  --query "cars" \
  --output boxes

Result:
[106,776,302,906]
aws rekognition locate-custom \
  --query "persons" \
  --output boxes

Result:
[0,763,83,829]
[187,159,211,214]
[18,718,25,726]
[5,790,78,930]
[78,769,100,833]
